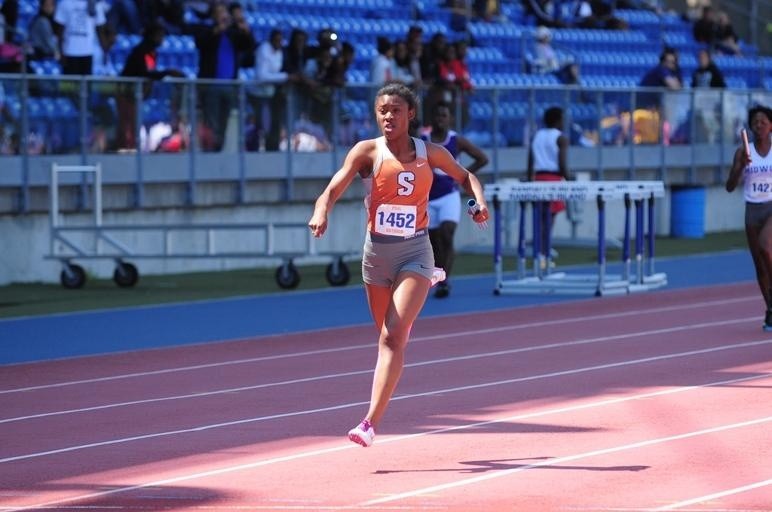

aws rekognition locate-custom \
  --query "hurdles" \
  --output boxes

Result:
[481,181,668,297]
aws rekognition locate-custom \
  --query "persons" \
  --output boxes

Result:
[725,105,772,332]
[417,100,488,297]
[306,83,489,446]
[529,108,574,271]
[0,0,746,152]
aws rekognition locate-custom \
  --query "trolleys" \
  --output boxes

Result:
[41,161,359,290]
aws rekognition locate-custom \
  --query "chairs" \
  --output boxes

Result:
[3,0,769,144]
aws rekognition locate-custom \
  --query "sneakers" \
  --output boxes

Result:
[348,421,376,448]
[429,267,449,298]
[541,260,555,268]
[762,310,772,331]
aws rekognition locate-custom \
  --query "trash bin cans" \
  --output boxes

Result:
[668,182,707,242]
